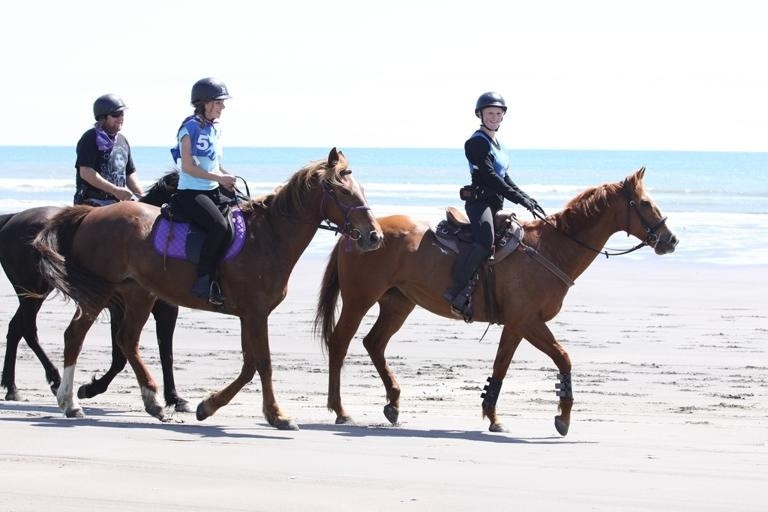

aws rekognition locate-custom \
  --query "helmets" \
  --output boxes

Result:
[475,91,507,115]
[191,77,231,104]
[94,94,127,119]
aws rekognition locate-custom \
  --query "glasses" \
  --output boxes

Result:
[109,111,124,118]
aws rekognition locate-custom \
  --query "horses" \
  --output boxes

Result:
[314,166,680,435]
[35,146,384,431]
[0,170,191,413]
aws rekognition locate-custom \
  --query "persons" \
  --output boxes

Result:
[74,94,145,207]
[170,77,237,301]
[444,92,537,322]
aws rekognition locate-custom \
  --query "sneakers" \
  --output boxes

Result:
[190,277,228,301]
[443,285,472,313]
